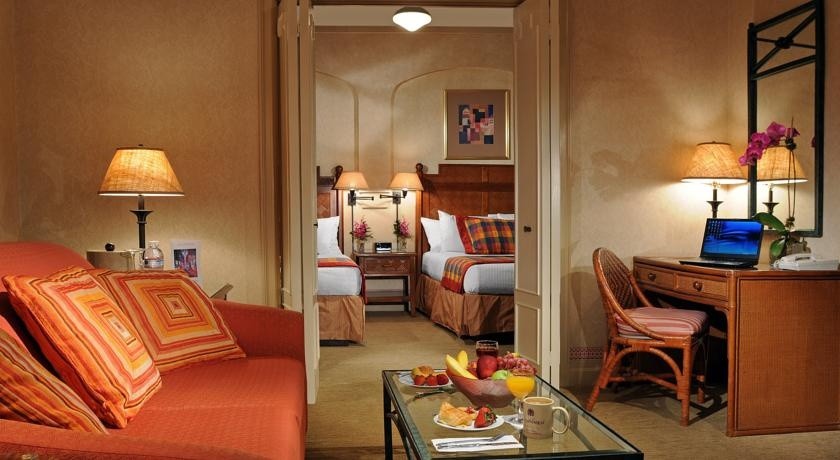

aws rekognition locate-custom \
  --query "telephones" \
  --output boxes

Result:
[778,252,840,271]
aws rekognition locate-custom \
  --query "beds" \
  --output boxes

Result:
[412,161,516,343]
[314,165,368,345]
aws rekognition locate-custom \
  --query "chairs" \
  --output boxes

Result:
[585,247,709,425]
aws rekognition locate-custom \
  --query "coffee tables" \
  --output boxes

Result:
[380,368,646,460]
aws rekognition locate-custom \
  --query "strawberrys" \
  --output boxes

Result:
[474,404,497,428]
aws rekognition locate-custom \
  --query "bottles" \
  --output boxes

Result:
[141,241,163,271]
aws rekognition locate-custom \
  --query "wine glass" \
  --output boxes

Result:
[505,366,535,428]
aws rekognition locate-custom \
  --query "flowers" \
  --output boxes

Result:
[393,216,412,249]
[740,112,810,264]
[351,216,374,249]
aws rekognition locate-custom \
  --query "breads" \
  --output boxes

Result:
[439,401,477,426]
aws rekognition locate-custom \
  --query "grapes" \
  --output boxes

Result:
[496,350,538,375]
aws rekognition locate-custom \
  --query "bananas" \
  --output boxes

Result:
[445,351,478,380]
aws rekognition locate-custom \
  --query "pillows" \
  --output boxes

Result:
[456,215,473,253]
[316,214,343,255]
[421,217,441,253]
[463,216,516,255]
[0,314,110,437]
[86,267,247,375]
[1,265,163,429]
[438,208,465,253]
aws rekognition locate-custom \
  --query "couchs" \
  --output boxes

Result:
[0,239,309,460]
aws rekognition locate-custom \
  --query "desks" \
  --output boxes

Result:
[630,254,839,437]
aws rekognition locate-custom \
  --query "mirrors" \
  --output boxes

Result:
[747,0,824,239]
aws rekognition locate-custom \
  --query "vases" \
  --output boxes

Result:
[356,240,366,253]
[398,236,408,252]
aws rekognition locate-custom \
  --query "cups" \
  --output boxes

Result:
[522,397,570,439]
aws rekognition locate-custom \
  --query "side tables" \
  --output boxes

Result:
[350,249,418,316]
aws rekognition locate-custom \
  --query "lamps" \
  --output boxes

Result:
[380,171,424,247]
[391,7,431,33]
[757,146,808,228]
[680,138,748,215]
[98,143,186,257]
[334,170,373,251]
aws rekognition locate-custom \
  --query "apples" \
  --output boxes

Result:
[477,355,513,380]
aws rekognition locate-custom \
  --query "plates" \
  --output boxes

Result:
[399,372,453,389]
[433,413,505,432]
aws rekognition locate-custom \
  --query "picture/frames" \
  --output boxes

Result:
[443,87,510,160]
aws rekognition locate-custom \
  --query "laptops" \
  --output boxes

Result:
[679,217,765,269]
